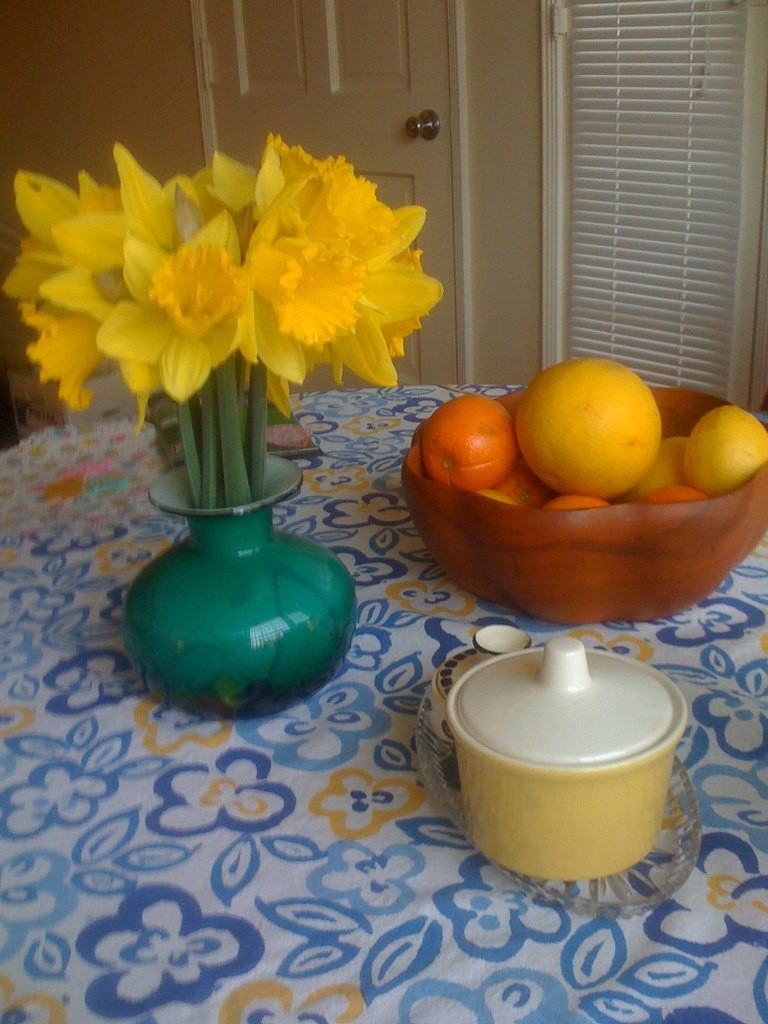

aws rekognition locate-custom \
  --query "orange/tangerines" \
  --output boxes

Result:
[419,394,707,509]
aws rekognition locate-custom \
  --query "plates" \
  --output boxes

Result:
[415,643,702,920]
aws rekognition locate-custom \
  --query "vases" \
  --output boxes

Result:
[120,452,359,720]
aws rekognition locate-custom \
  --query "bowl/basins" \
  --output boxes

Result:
[401,385,767,626]
[445,634,688,879]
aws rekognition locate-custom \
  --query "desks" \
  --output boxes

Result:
[0,384,768,1024]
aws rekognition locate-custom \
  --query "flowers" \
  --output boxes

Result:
[2,129,445,507]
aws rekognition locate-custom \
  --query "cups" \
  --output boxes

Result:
[472,626,530,662]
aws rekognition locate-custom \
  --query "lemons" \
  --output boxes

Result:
[516,359,768,498]
